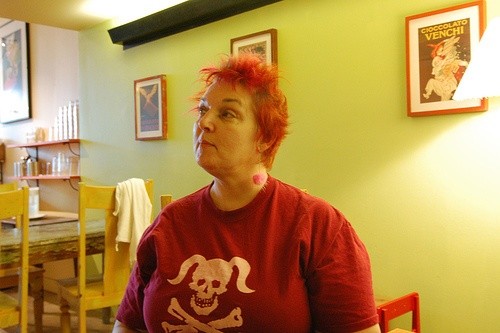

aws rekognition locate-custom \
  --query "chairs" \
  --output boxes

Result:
[0,178,153,333]
[375,293,421,333]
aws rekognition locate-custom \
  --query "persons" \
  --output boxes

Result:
[111,50,382,333]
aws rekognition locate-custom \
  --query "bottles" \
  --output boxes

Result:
[47,99,80,141]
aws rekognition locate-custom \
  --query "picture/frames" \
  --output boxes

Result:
[0,19,32,124]
[230,28,278,70]
[134,74,167,142]
[405,0,489,117]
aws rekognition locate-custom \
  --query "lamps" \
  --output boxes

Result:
[106,0,283,52]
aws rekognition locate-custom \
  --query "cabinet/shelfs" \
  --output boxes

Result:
[6,139,81,179]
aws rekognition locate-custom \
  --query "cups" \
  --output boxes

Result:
[14,152,72,177]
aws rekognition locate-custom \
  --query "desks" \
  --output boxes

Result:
[0,217,107,270]
